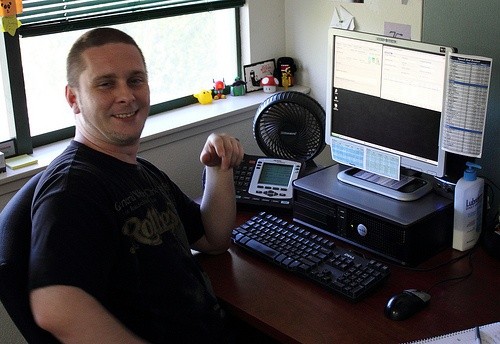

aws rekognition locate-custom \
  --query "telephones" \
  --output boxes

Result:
[202,154,304,215]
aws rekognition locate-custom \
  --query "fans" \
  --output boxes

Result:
[252,90,326,170]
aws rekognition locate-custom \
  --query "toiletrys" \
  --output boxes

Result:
[453,161,485,252]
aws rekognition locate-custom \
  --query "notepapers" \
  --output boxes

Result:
[5,153,37,170]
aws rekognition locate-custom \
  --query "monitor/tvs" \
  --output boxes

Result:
[324,27,458,178]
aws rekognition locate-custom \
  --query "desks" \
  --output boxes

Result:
[190,210,500,344]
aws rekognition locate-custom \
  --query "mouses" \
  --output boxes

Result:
[384,288,432,321]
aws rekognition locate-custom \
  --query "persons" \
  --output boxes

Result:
[29,28,244,344]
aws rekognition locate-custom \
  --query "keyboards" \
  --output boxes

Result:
[230,211,392,304]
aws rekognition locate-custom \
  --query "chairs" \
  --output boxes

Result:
[0,168,62,344]
